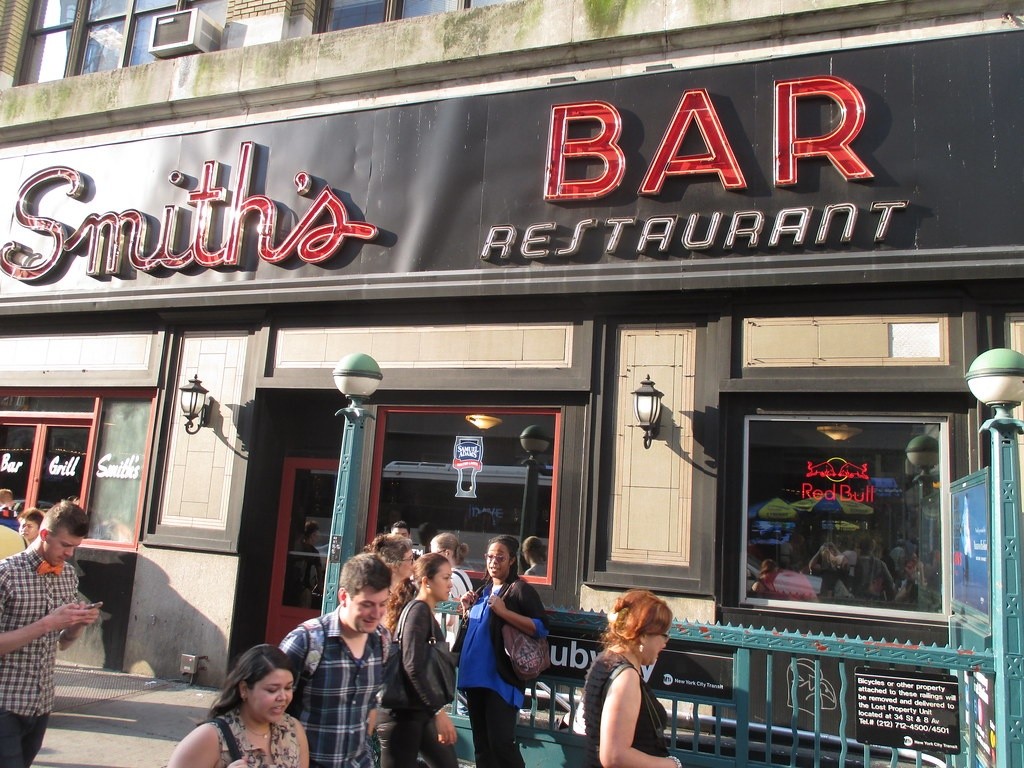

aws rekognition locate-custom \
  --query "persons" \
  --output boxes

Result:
[457,536,549,768]
[752,559,778,599]
[417,522,438,553]
[522,536,547,577]
[299,521,321,610]
[583,589,682,768]
[809,537,920,604]
[278,553,401,768]
[0,483,103,768]
[363,521,474,768]
[166,644,309,768]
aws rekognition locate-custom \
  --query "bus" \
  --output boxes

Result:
[377,460,552,578]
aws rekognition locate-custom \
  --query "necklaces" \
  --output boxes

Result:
[244,725,272,739]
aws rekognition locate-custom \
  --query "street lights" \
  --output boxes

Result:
[515,423,552,578]
[903,434,939,611]
[319,352,383,619]
[963,347,1024,768]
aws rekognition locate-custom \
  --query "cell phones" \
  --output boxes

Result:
[83,601,103,610]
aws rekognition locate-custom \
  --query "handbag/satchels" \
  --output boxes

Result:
[496,579,550,680]
[375,600,459,712]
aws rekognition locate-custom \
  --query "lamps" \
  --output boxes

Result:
[815,425,861,442]
[630,375,664,447]
[179,374,210,434]
[465,413,501,431]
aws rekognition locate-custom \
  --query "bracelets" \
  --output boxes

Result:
[665,756,682,768]
[435,708,444,715]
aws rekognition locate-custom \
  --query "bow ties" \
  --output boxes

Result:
[37,560,65,577]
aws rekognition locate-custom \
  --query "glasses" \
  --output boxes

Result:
[655,632,672,642]
[483,552,511,564]
[399,551,418,561]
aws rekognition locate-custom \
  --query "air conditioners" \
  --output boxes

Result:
[146,9,221,57]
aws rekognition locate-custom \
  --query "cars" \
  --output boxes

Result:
[0,498,57,515]
